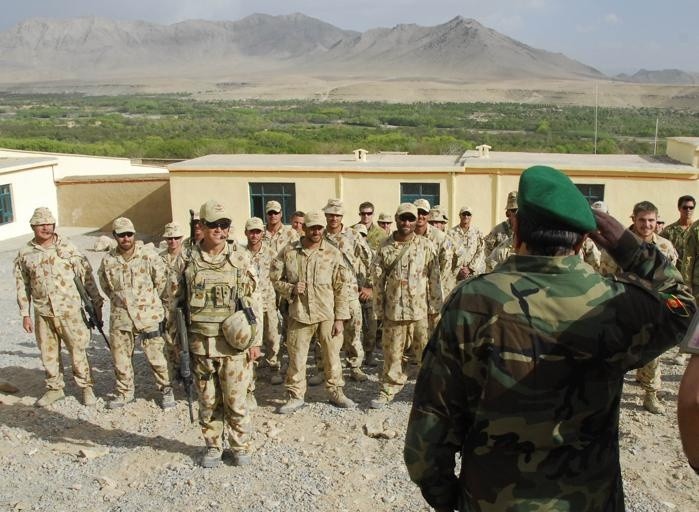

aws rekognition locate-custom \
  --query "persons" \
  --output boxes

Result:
[153,222,187,393]
[660,195,697,272]
[428,203,467,298]
[182,213,207,259]
[97,217,177,413]
[399,198,457,383]
[447,206,486,285]
[376,210,395,238]
[349,201,387,349]
[242,216,284,386]
[486,209,518,270]
[576,234,599,269]
[684,218,699,309]
[340,224,384,368]
[260,200,301,351]
[12,208,104,409]
[290,210,309,233]
[676,306,699,479]
[628,212,639,230]
[168,199,264,468]
[599,200,678,416]
[368,203,442,409]
[269,210,356,415]
[656,212,666,234]
[306,198,378,387]
[482,189,520,256]
[402,165,698,512]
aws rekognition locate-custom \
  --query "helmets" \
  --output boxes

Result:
[220,309,261,352]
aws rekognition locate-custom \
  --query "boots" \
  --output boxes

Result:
[201,446,224,468]
[365,351,377,366]
[276,398,305,413]
[308,370,326,386]
[269,367,282,386]
[108,393,134,409]
[36,389,66,407]
[230,447,249,466]
[350,367,367,382]
[643,391,665,414]
[161,392,175,409]
[367,390,390,409]
[81,387,95,406]
[329,388,354,409]
[673,352,686,364]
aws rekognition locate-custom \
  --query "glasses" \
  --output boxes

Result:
[461,213,471,216]
[360,212,373,216]
[117,233,132,237]
[682,206,694,210]
[206,221,229,228]
[398,214,415,222]
[167,237,180,240]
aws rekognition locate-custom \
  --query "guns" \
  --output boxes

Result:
[181,206,196,314]
[159,315,166,337]
[173,303,195,424]
[274,267,289,343]
[74,273,112,351]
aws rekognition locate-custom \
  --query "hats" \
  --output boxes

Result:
[265,200,281,214]
[396,203,418,218]
[304,210,326,228]
[192,211,200,220]
[504,191,518,210]
[377,213,392,223]
[517,165,598,232]
[460,207,472,215]
[656,215,664,223]
[427,209,448,223]
[414,200,430,213]
[112,217,136,234]
[323,198,344,215]
[591,201,608,214]
[199,200,232,223]
[354,225,368,236]
[428,205,447,220]
[162,222,184,238]
[245,217,264,231]
[29,207,56,225]
